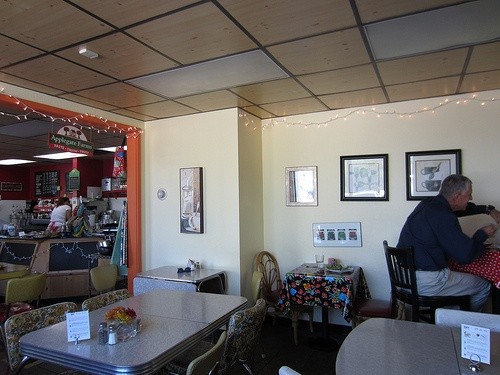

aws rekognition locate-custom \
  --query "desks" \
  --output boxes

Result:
[17,290,248,375]
[275,263,373,347]
[336,318,500,375]
[136,265,226,292]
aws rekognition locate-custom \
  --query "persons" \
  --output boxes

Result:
[50,197,72,233]
[396,175,500,313]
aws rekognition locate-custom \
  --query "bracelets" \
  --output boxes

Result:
[487,205,494,215]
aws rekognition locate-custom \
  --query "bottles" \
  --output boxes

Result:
[196,262,200,269]
[8,224,15,236]
[316,253,325,268]
[108,325,118,345]
[191,262,195,271]
[97,322,108,344]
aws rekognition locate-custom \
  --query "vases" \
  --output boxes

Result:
[112,320,137,340]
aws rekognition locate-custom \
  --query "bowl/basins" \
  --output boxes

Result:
[99,247,112,255]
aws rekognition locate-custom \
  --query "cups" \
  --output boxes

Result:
[328,258,335,265]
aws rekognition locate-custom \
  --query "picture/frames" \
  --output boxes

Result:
[285,166,318,207]
[340,154,389,201]
[405,149,461,201]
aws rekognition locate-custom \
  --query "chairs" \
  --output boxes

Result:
[0,250,313,375]
[353,240,500,333]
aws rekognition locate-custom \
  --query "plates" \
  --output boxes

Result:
[326,267,353,273]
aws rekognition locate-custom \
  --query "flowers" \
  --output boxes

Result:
[105,305,137,324]
